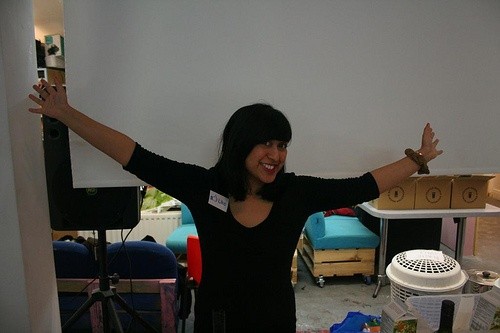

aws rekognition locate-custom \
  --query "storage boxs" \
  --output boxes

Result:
[369,173,496,209]
[45,34,65,69]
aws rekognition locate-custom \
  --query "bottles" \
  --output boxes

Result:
[434,299,455,333]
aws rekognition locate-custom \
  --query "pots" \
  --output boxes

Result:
[462,270,499,295]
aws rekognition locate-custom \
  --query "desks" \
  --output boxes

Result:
[358,202,500,297]
[76,211,183,244]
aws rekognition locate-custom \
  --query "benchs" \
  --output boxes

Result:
[300,210,379,286]
[168,200,297,287]
[52,240,183,333]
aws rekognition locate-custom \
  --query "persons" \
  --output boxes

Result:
[28,75,444,333]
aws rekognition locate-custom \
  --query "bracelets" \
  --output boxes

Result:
[404,148,430,174]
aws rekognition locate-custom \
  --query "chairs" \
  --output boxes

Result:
[182,235,203,333]
